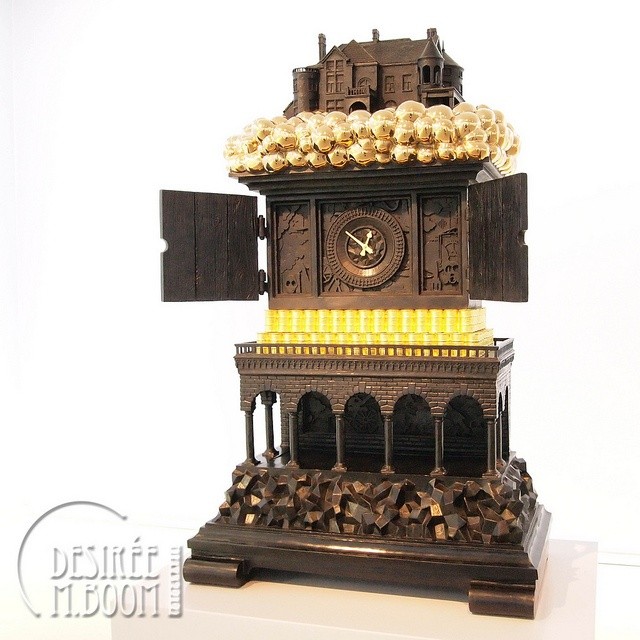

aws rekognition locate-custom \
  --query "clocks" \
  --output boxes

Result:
[327,206,406,286]
